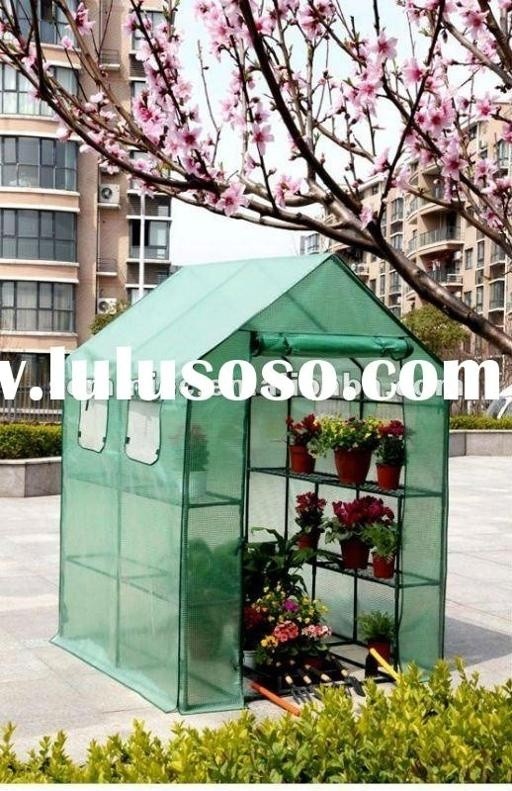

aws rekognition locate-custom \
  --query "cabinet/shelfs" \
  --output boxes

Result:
[65,471,241,609]
[249,465,441,588]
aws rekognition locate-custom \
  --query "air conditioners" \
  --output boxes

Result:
[96,298,118,316]
[99,184,121,204]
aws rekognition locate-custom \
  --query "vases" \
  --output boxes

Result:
[242,651,259,670]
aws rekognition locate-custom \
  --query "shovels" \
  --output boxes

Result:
[304,664,347,696]
[330,655,366,697]
[297,669,324,700]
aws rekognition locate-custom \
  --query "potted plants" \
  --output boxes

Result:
[357,608,400,666]
